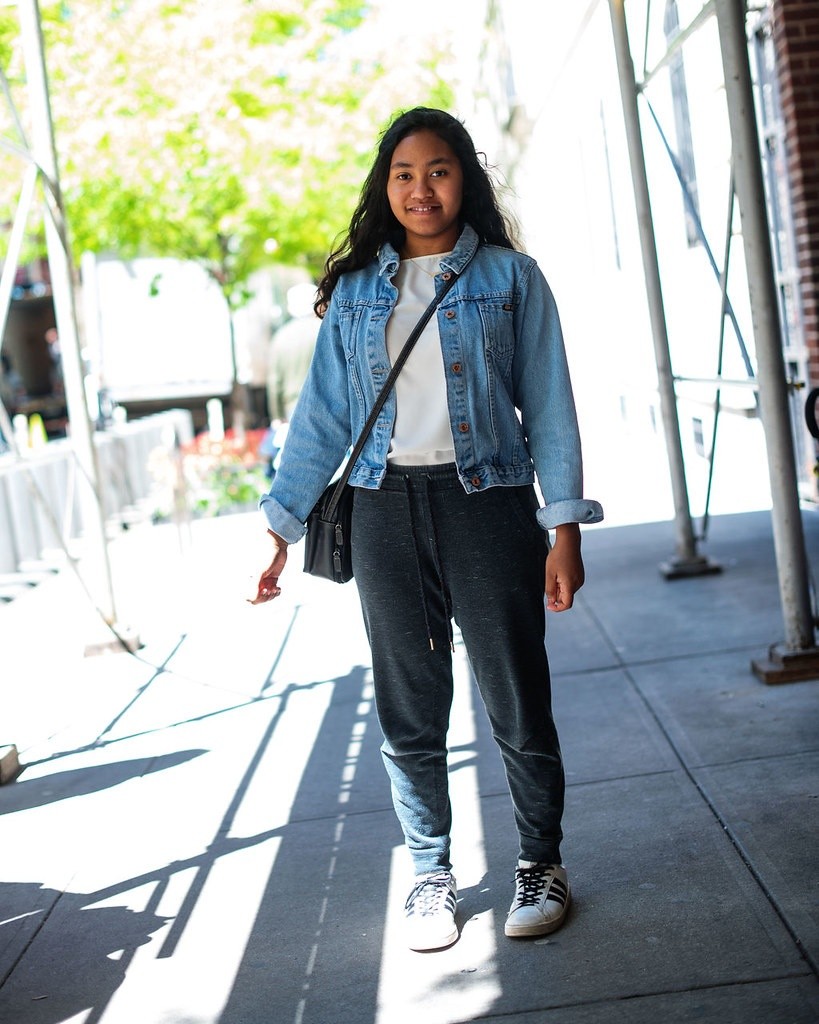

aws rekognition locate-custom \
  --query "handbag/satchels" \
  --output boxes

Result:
[305,477,354,586]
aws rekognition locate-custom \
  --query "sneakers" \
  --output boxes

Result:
[406,875,461,950]
[501,858,573,938]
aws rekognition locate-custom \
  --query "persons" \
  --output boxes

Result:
[237,104,606,955]
